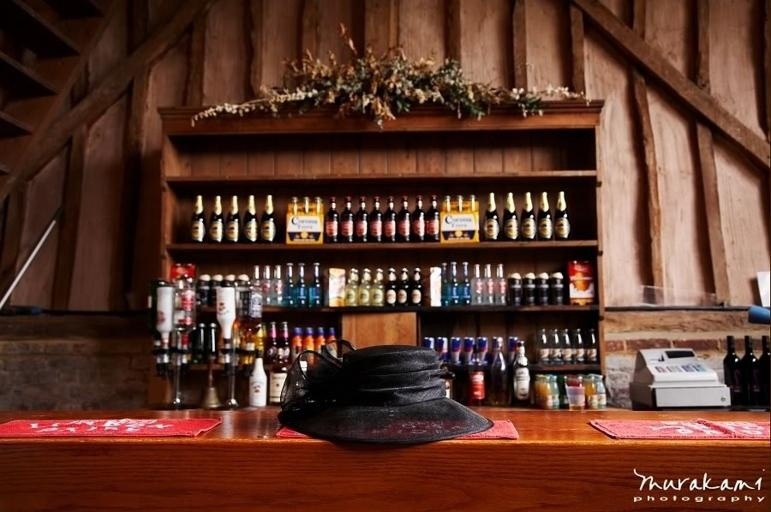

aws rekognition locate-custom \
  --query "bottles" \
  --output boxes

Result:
[173,260,567,311]
[245,349,289,408]
[536,371,609,411]
[724,331,771,406]
[422,335,531,408]
[188,188,572,248]
[214,286,237,363]
[535,325,599,364]
[161,316,338,367]
[145,278,175,365]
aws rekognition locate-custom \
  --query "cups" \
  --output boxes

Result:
[747,304,771,325]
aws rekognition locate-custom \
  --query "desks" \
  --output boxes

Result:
[2,391,770,509]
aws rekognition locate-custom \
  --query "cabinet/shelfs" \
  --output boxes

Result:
[133,74,617,412]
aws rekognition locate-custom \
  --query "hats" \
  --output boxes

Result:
[278,339,494,445]
